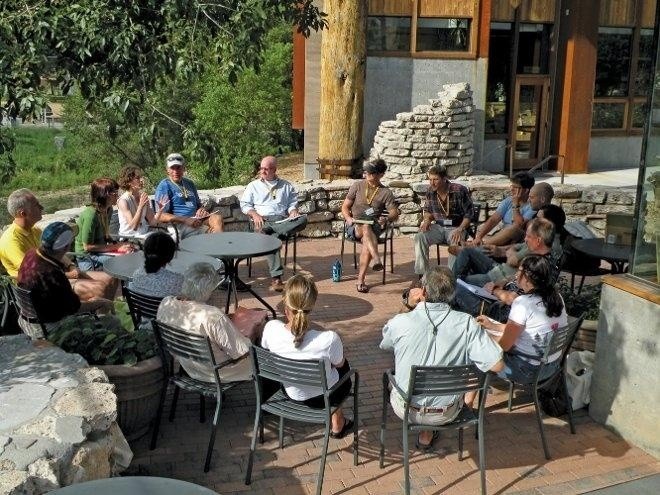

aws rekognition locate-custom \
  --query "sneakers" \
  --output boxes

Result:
[218,282,250,291]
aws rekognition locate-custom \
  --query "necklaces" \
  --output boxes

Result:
[170,177,187,198]
[267,186,276,199]
[424,302,452,336]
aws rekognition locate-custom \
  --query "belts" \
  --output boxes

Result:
[412,407,443,413]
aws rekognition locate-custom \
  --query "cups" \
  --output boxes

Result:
[503,96,506,100]
[498,96,503,101]
[608,235,615,244]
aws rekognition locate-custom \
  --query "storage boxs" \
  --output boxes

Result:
[606,211,635,244]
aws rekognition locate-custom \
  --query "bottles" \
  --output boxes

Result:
[332,259,341,282]
[524,63,540,73]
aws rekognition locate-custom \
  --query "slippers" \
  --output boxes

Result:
[356,284,369,293]
[330,418,354,439]
[373,263,383,271]
[415,430,439,450]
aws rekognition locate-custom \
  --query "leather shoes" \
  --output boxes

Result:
[271,276,284,292]
[260,226,276,235]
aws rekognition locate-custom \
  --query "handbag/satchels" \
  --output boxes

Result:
[540,378,568,418]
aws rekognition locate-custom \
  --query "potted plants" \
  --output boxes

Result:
[47,299,165,441]
[555,277,602,354]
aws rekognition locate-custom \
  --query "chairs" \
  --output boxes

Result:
[341,200,399,285]
[66,250,95,273]
[109,210,170,250]
[379,364,490,495]
[123,286,164,331]
[245,345,361,495]
[487,249,568,317]
[247,219,297,277]
[150,319,264,473]
[437,204,481,265]
[563,216,615,295]
[3,275,99,339]
[150,199,179,242]
[475,314,584,461]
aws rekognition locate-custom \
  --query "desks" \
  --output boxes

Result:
[103,250,225,296]
[43,477,221,495]
[571,239,656,293]
[178,232,282,319]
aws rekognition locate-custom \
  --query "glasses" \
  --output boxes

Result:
[167,156,183,162]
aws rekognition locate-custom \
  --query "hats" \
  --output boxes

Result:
[363,159,387,173]
[167,153,185,168]
[41,221,79,251]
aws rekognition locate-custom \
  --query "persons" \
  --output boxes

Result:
[341,160,398,292]
[155,153,223,241]
[465,254,567,407]
[134,232,184,329]
[400,165,566,324]
[262,274,352,440]
[380,263,504,450]
[2,188,118,315]
[75,177,140,273]
[157,262,280,405]
[118,166,170,240]
[241,156,307,291]
[17,223,120,339]
